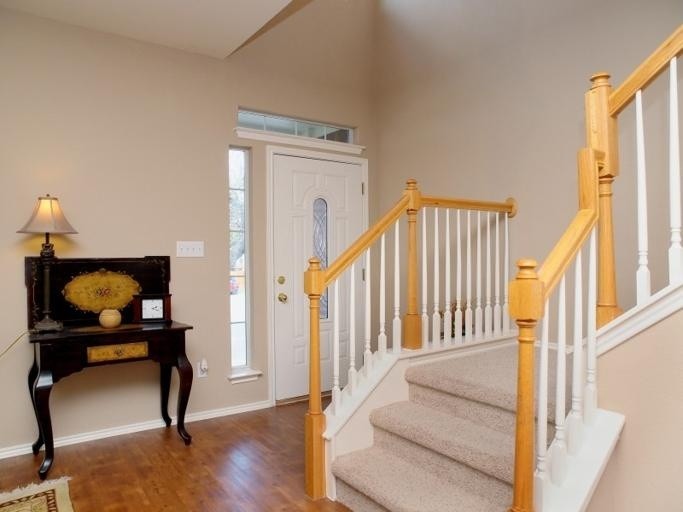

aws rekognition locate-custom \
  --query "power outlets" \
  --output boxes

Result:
[197,362,207,378]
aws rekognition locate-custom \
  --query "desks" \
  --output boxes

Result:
[24,257,194,479]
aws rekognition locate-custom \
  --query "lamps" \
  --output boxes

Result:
[15,194,79,332]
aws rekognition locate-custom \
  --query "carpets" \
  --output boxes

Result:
[0,475,75,512]
